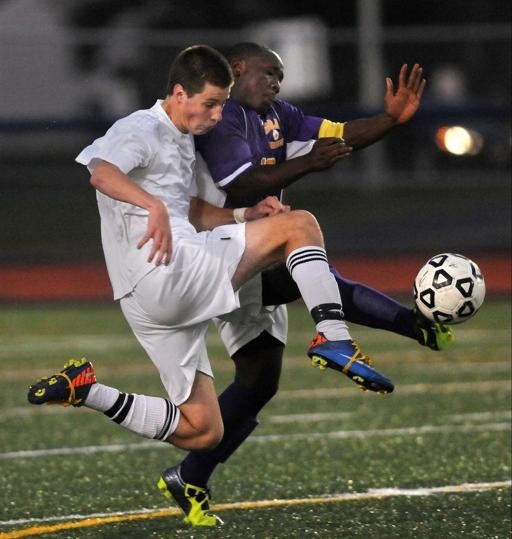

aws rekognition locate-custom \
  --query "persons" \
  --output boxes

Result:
[28,43,395,455]
[152,38,456,530]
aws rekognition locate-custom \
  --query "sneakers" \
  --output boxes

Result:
[27,356,97,408]
[307,331,394,395]
[414,306,457,352]
[157,465,224,527]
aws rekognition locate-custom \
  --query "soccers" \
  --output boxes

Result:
[414,253,485,323]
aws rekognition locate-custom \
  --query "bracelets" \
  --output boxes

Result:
[232,207,250,225]
